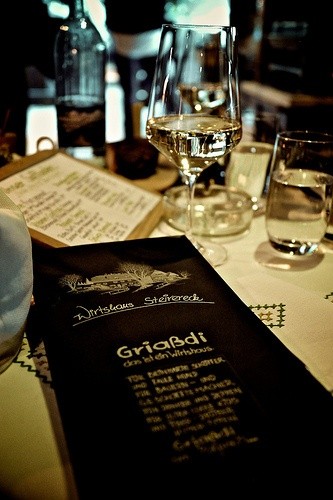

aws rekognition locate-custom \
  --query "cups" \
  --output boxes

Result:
[266,130,333,261]
[224,142,280,199]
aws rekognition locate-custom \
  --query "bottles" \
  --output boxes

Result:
[54,0,108,161]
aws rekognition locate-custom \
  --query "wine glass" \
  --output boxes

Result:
[146,23,244,268]
